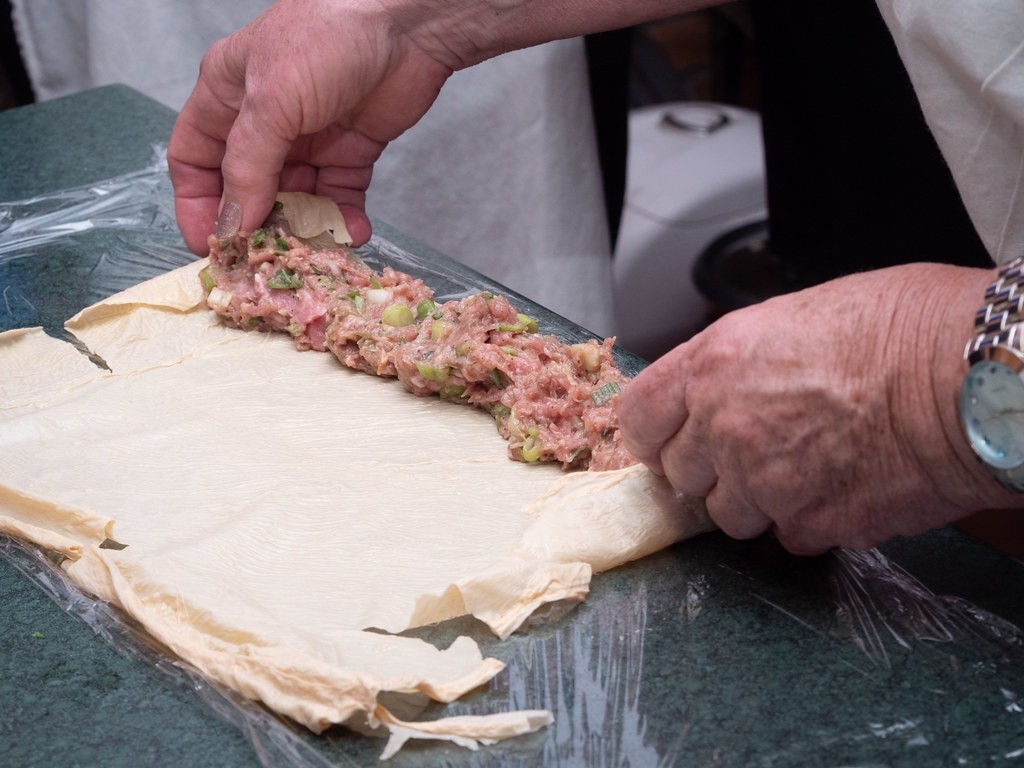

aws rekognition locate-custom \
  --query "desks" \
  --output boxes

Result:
[0,81,1024,768]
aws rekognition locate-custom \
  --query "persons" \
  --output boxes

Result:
[165,0,1024,555]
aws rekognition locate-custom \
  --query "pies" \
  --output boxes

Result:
[0,193,715,760]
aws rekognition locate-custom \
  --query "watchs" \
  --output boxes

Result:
[957,254,1024,492]
